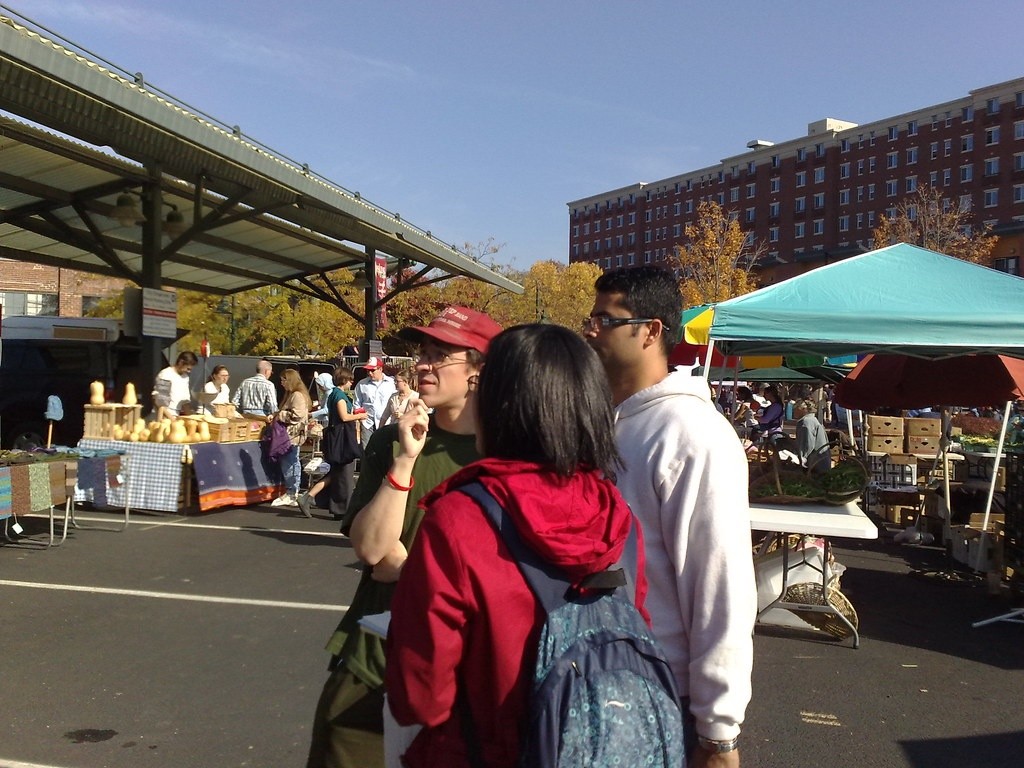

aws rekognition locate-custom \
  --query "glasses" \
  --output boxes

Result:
[367,369,378,372]
[394,379,404,383]
[412,348,467,365]
[582,316,669,331]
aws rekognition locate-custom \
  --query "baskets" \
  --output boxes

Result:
[745,431,866,506]
[782,573,858,642]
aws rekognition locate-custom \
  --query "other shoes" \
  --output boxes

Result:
[271,493,298,507]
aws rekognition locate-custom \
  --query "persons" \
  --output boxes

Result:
[708,382,838,471]
[384,323,652,767]
[296,357,432,519]
[908,399,1024,437]
[231,361,278,415]
[584,266,757,767]
[307,306,504,768]
[201,365,230,415]
[150,351,199,420]
[266,369,311,507]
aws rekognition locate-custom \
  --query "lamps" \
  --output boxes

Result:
[161,201,187,240]
[108,187,149,227]
[352,267,372,291]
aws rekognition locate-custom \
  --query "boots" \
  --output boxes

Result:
[297,493,316,518]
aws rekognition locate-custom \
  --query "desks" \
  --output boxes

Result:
[0,459,67,550]
[5,446,134,547]
[77,437,275,513]
[750,502,878,649]
[968,451,1008,490]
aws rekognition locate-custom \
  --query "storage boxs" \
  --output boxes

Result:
[877,503,1003,589]
[865,415,955,503]
[1003,447,1024,597]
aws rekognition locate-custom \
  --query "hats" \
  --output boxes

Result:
[363,356,383,370]
[399,305,504,357]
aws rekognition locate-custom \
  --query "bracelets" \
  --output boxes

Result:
[387,471,414,491]
[697,736,739,752]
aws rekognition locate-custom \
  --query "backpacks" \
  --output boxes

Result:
[461,482,686,768]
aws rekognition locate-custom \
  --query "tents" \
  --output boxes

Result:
[677,242,1024,573]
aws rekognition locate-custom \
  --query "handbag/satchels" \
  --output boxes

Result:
[322,420,360,465]
